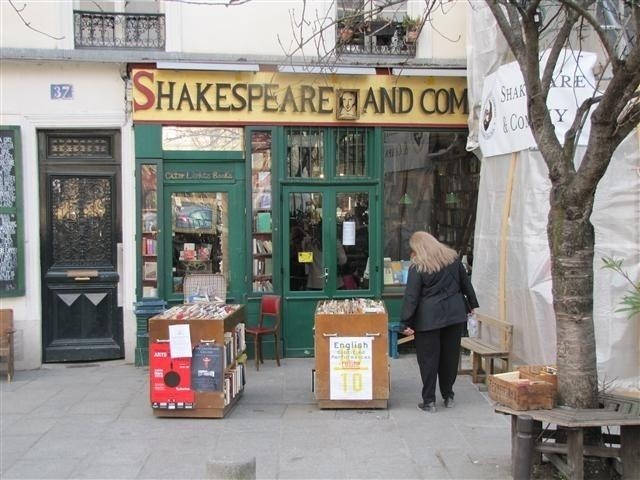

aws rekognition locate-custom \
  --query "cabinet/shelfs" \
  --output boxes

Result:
[147,299,247,420]
[313,297,391,410]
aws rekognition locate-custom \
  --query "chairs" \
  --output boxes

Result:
[247,294,282,371]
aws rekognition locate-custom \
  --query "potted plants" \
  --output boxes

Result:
[401,13,422,43]
[339,9,362,43]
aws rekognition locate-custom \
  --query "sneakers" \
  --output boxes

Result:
[417,400,437,414]
[443,397,455,408]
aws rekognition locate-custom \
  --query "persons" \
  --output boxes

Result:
[288,214,370,291]
[400,230,480,414]
[383,218,413,261]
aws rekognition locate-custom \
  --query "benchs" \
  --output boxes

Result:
[495,395,640,480]
[460,312,514,391]
